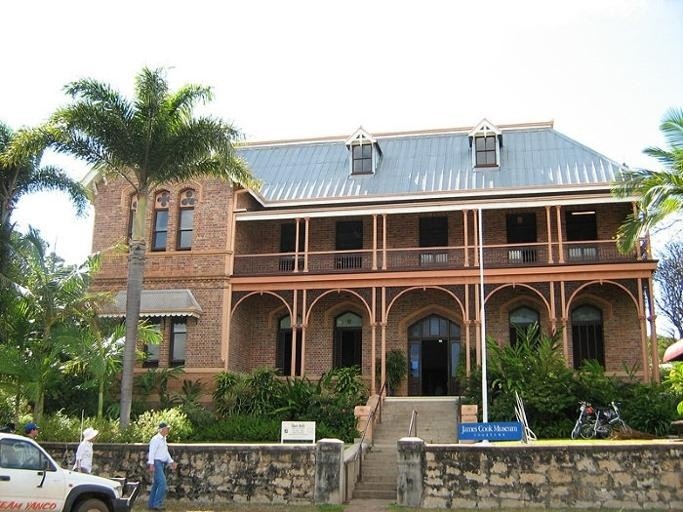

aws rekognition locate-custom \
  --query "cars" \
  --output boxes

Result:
[0,433,141,512]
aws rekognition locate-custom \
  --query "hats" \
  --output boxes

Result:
[158,422,170,429]
[24,423,40,430]
[83,427,98,441]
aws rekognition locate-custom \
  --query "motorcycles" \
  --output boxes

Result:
[570,400,632,441]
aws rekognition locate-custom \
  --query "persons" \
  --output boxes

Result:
[23,423,40,441]
[147,423,176,512]
[72,427,100,474]
